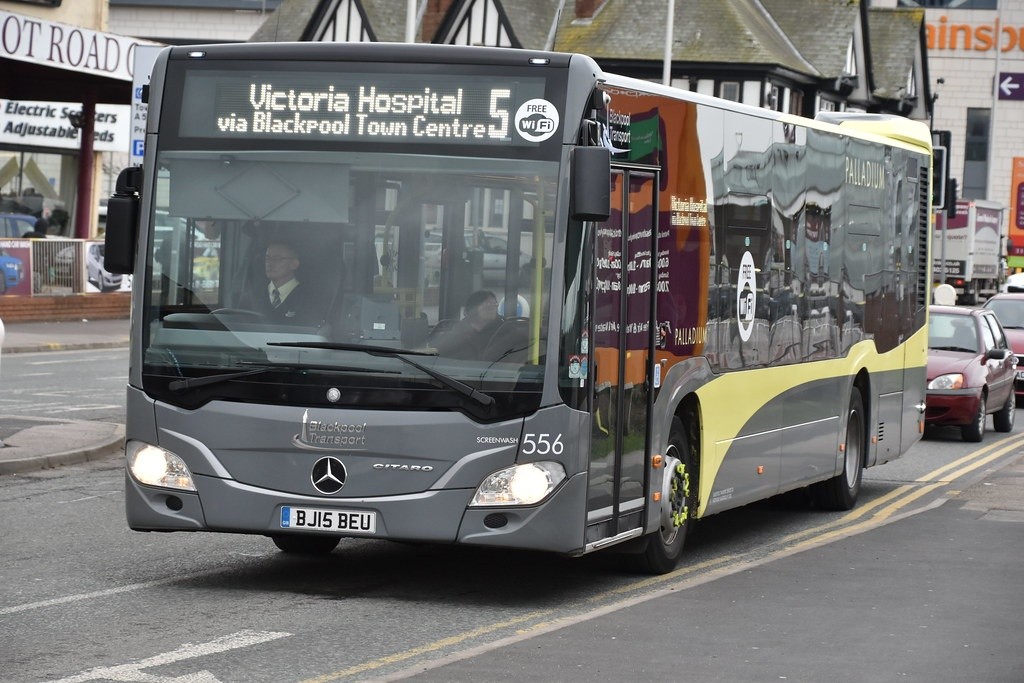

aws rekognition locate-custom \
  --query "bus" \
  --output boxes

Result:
[102,42,934,576]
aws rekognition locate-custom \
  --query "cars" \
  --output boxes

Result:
[0,250,23,296]
[54,244,76,283]
[190,242,221,289]
[152,247,162,291]
[924,305,1019,442]
[462,232,547,283]
[985,292,1024,409]
[423,232,442,269]
[0,212,40,238]
[86,242,122,291]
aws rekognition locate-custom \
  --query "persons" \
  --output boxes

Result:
[455,290,507,362]
[22,218,47,294]
[238,239,329,327]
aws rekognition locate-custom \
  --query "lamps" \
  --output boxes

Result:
[69,109,85,127]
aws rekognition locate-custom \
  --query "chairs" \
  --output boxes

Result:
[481,317,545,364]
[237,259,346,321]
[429,318,458,350]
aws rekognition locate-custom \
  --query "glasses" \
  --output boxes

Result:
[265,254,281,264]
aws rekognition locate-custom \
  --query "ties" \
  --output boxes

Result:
[272,289,281,308]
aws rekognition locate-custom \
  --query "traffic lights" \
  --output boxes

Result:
[946,277,965,286]
[934,146,946,211]
